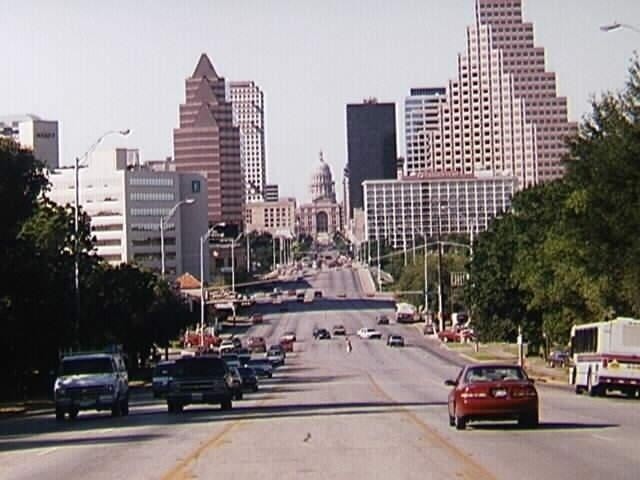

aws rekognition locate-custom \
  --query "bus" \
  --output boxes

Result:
[567,317,639,399]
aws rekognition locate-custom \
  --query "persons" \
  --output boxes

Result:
[346,337,352,352]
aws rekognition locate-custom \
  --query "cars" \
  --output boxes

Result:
[422,318,470,341]
[445,360,538,429]
[53,352,129,421]
[253,287,296,324]
[295,291,305,303]
[549,351,568,368]
[151,331,297,411]
[314,291,321,296]
[313,302,415,347]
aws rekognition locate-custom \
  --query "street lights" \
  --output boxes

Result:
[75,127,132,295]
[599,21,639,34]
[430,195,445,335]
[201,221,226,318]
[160,199,198,273]
